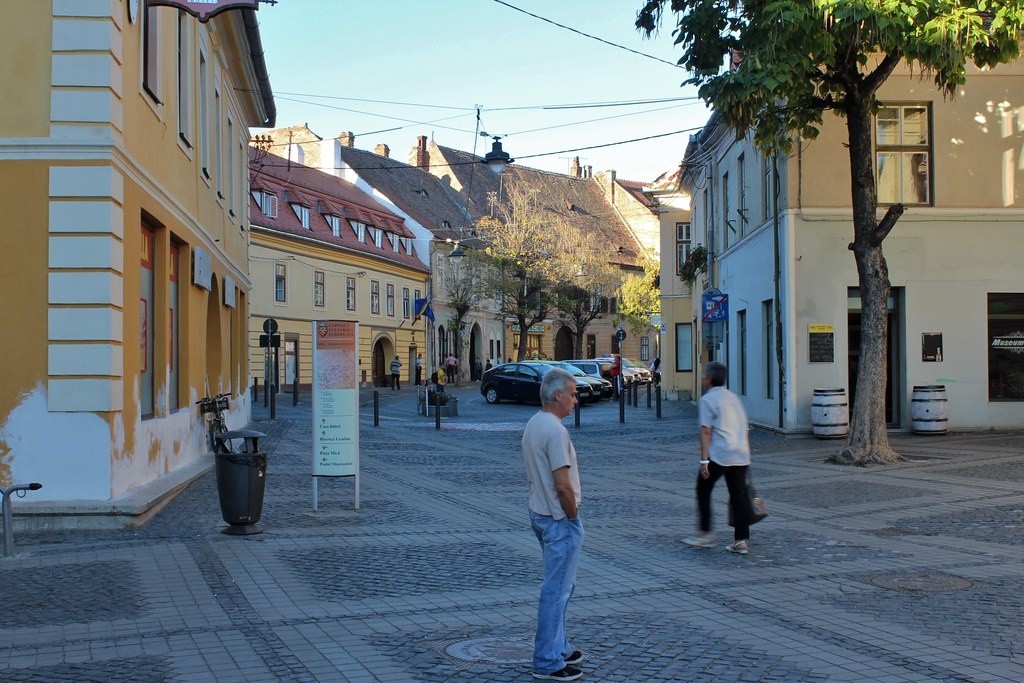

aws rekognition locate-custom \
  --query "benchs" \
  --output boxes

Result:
[501,370,515,376]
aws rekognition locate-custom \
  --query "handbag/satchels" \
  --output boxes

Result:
[729,486,769,527]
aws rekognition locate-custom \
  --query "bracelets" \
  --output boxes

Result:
[700,460,710,464]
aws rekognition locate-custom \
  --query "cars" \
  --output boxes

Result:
[481,363,593,404]
[522,354,661,398]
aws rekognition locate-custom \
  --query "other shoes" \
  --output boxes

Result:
[532,664,583,681]
[476,380,481,382]
[564,650,584,665]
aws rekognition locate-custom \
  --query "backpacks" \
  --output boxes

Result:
[432,371,440,383]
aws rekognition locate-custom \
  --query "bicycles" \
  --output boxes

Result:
[195,393,233,453]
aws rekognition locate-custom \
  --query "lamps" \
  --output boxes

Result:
[481,135,515,173]
[447,241,467,264]
[574,263,588,280]
[358,271,366,277]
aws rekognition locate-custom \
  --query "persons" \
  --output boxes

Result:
[485,359,492,373]
[521,368,585,681]
[508,358,512,364]
[432,362,448,396]
[474,358,483,382]
[390,356,402,390]
[650,358,661,390]
[610,356,620,401]
[414,354,422,386]
[497,354,502,366]
[681,362,751,553]
[444,353,458,383]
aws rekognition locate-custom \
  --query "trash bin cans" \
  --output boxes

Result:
[215,429,268,536]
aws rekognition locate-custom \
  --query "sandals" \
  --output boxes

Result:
[728,539,748,554]
[681,532,718,547]
